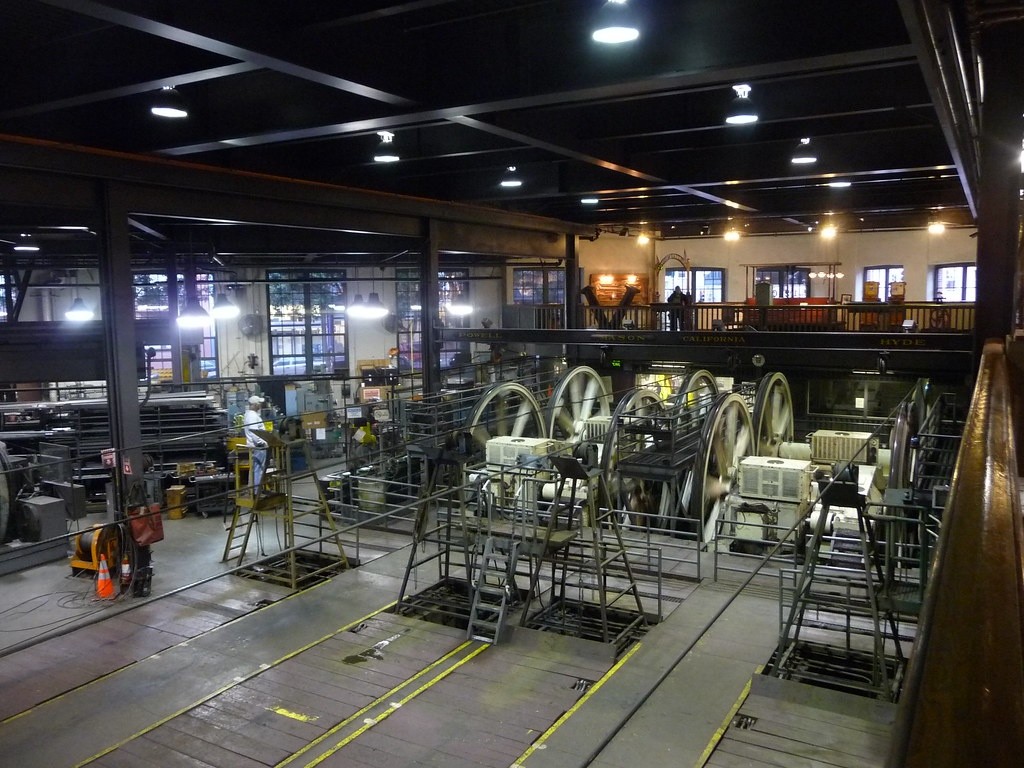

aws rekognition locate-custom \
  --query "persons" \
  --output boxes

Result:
[667,285,688,331]
[243,396,271,497]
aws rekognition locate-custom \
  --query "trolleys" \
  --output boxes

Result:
[188,474,237,519]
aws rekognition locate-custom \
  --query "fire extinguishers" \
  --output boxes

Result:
[121,554,131,585]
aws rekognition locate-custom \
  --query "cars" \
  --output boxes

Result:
[273,356,326,375]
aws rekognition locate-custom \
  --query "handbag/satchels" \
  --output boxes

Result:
[124,482,164,547]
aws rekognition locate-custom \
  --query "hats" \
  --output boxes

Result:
[248,396,265,404]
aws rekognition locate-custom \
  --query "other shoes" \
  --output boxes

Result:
[258,493,266,498]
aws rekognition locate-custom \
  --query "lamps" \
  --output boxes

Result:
[445,265,474,315]
[57,250,95,325]
[592,0,639,44]
[830,177,852,187]
[902,319,921,334]
[712,319,724,332]
[481,318,494,328]
[173,228,210,330]
[726,84,758,124]
[499,161,525,187]
[151,85,191,118]
[792,137,818,163]
[623,320,636,332]
[373,131,399,163]
[207,254,240,320]
[346,265,391,320]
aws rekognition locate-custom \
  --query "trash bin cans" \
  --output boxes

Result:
[290,449,307,471]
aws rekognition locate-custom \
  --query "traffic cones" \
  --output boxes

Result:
[91,553,119,601]
